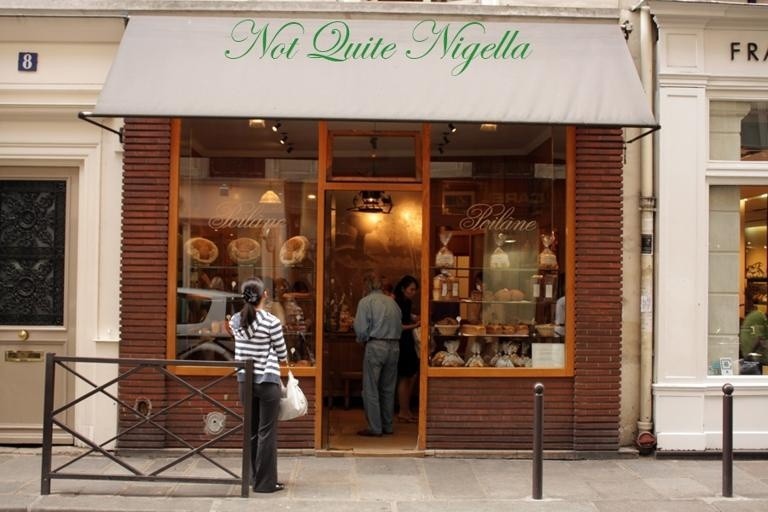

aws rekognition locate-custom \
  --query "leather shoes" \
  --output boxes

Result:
[257,483,284,493]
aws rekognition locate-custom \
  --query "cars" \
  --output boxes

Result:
[176,287,244,360]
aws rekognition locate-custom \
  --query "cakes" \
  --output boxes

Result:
[460,319,486,335]
[488,322,529,335]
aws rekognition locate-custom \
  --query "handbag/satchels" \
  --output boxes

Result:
[276,370,310,420]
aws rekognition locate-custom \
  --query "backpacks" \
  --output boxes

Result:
[434,323,459,335]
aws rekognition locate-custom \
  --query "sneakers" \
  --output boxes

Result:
[357,427,394,436]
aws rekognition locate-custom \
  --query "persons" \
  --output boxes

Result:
[350,268,402,437]
[261,275,316,363]
[227,274,291,495]
[389,275,419,423]
[472,272,505,344]
[199,263,225,320]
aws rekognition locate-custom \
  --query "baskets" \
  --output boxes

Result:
[463,324,484,335]
[536,323,559,337]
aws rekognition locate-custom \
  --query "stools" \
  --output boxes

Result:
[326,365,360,411]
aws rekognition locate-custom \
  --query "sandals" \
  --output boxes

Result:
[396,413,418,424]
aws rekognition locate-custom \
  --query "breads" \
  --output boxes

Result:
[432,278,460,302]
[283,239,302,259]
[191,238,214,260]
[235,239,257,259]
[494,287,525,301]
[436,317,458,325]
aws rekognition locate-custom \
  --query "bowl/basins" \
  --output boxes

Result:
[535,326,555,336]
[748,356,762,362]
[434,325,488,336]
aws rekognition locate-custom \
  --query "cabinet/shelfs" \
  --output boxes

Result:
[430,266,565,368]
[177,261,315,366]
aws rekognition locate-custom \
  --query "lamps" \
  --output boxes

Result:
[354,137,392,216]
[258,180,282,205]
[439,123,458,154]
[271,122,295,155]
